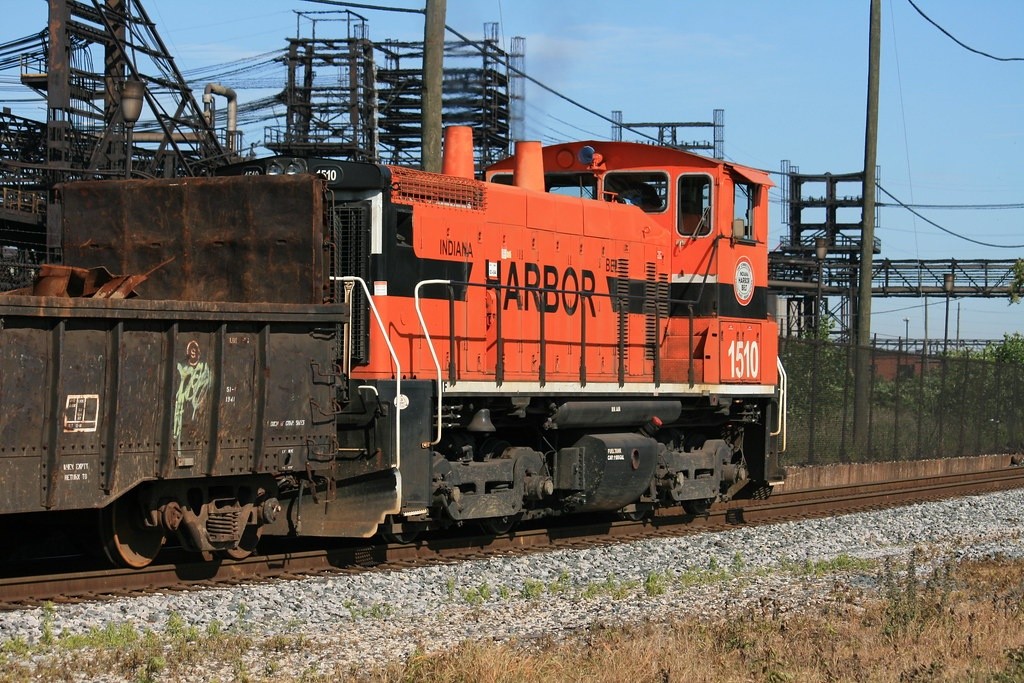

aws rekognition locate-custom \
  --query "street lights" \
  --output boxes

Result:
[807,238,828,466]
[115,80,146,177]
[937,274,955,459]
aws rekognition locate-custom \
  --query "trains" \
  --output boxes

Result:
[0,125,787,569]
